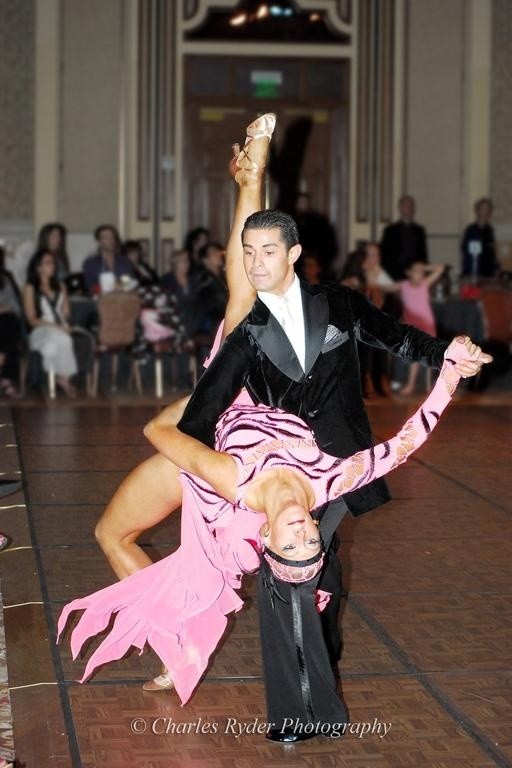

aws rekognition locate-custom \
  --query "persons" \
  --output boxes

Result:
[178,208,493,746]
[90,109,497,690]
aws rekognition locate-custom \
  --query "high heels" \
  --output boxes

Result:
[227,112,277,184]
[0,378,24,400]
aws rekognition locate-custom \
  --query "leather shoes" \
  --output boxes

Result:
[264,723,348,744]
[141,662,177,692]
[56,378,79,402]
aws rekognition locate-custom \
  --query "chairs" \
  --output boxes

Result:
[88,293,198,400]
